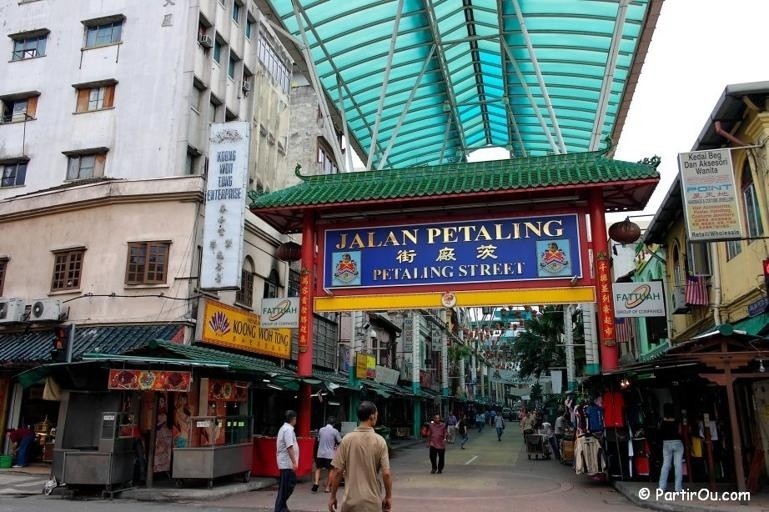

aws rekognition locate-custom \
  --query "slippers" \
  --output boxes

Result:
[312,484,318,491]
[325,488,331,493]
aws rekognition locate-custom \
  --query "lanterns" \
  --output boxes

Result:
[608,218,641,247]
[462,304,544,371]
[277,240,302,267]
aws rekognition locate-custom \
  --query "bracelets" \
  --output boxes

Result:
[444,438,447,440]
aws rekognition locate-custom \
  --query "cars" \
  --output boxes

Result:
[502,407,522,421]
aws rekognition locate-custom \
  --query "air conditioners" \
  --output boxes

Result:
[243,81,249,90]
[200,35,212,48]
[671,288,691,314]
[0,298,61,324]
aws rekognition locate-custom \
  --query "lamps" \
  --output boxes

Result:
[619,374,631,389]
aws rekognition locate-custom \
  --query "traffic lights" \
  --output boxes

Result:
[762,258,769,295]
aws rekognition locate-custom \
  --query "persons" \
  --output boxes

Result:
[146,392,167,429]
[328,400,393,512]
[655,403,686,492]
[5,428,35,468]
[274,409,300,512]
[335,254,357,271]
[444,406,567,462]
[543,242,565,262]
[311,416,342,492]
[174,393,195,447]
[426,414,448,474]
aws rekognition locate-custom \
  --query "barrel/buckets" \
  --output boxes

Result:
[1,454,13,468]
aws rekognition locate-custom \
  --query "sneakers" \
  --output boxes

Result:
[11,464,27,468]
[431,469,441,473]
[658,490,686,495]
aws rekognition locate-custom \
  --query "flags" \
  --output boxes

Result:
[684,274,709,306]
[614,317,630,343]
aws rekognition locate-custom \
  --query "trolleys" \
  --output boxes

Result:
[524,433,552,459]
